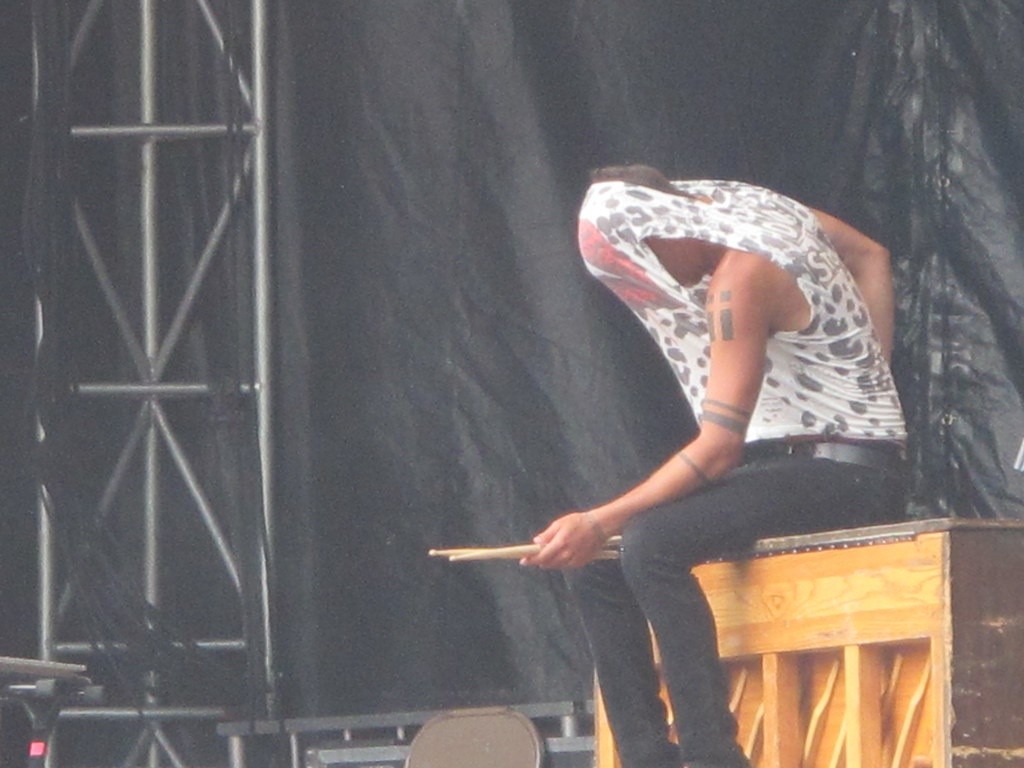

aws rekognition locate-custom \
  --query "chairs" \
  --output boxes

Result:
[402,707,547,768]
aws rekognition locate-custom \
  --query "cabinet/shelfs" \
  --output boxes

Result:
[591,516,1024,768]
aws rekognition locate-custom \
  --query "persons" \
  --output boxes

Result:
[518,165,910,768]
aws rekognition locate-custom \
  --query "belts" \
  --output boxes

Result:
[789,442,887,470]
